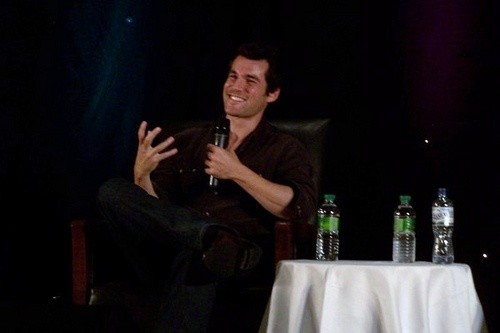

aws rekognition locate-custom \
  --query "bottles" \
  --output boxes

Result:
[392,196,416,262]
[432,188,455,264]
[316,194,340,261]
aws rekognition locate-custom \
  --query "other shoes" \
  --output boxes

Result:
[199,222,261,271]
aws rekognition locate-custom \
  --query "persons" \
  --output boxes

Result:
[97,42,321,333]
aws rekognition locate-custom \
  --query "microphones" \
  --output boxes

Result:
[209,118,230,187]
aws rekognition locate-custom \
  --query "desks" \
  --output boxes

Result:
[260,260,487,333]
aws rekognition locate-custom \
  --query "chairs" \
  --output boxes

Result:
[71,118,330,333]
